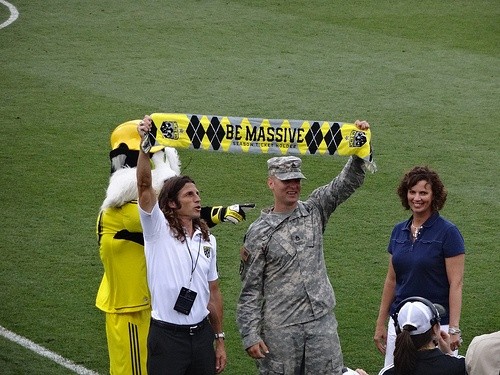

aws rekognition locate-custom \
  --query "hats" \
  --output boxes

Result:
[267,156,306,180]
[398,301,446,335]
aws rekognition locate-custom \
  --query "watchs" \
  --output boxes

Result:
[448,327,461,335]
[214,331,226,340]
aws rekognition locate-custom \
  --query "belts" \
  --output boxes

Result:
[151,317,207,335]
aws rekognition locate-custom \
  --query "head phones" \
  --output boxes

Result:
[394,296,441,335]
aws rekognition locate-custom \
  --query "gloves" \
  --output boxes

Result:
[211,203,255,225]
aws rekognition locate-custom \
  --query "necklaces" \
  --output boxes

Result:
[412,221,422,239]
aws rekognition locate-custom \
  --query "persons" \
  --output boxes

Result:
[95,120,256,375]
[137,114,227,375]
[236,120,377,375]
[374,166,466,367]
[355,297,467,375]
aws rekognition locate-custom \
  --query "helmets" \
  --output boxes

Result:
[111,120,165,153]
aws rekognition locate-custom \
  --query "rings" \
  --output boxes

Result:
[139,121,144,126]
